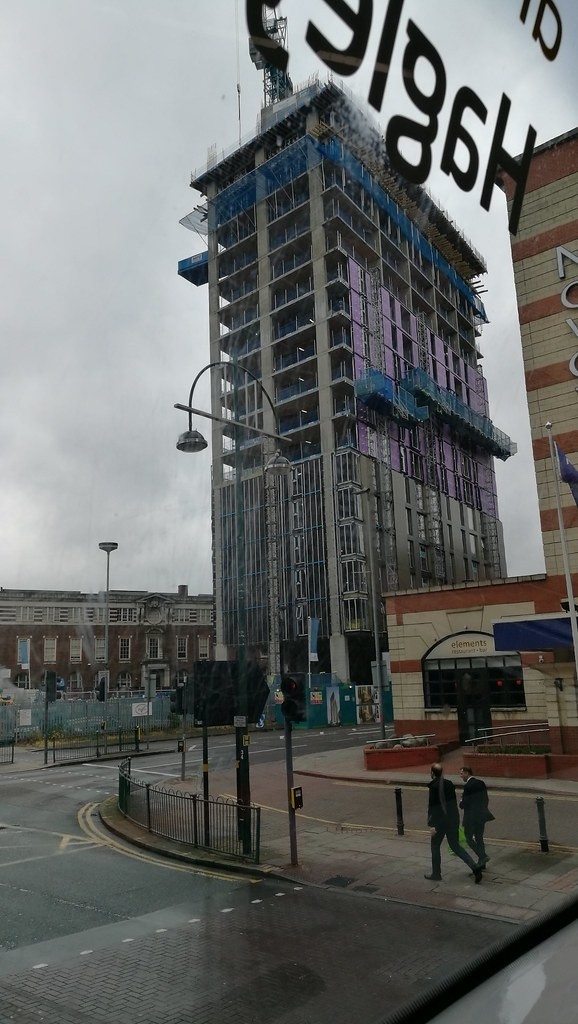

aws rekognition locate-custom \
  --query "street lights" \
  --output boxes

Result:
[98,542,119,753]
[173,360,294,854]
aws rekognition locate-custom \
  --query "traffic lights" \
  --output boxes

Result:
[101,721,106,730]
[290,786,304,809]
[278,670,307,722]
[46,670,61,702]
[170,686,184,715]
[177,740,185,752]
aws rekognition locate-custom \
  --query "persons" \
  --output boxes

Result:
[459,767,496,870]
[424,763,483,884]
[57,674,65,698]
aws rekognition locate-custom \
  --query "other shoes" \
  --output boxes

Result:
[473,865,482,883]
[475,856,490,869]
[424,874,442,881]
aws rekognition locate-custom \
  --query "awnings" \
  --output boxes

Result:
[492,617,578,652]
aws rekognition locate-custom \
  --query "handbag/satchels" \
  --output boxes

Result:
[447,826,465,855]
